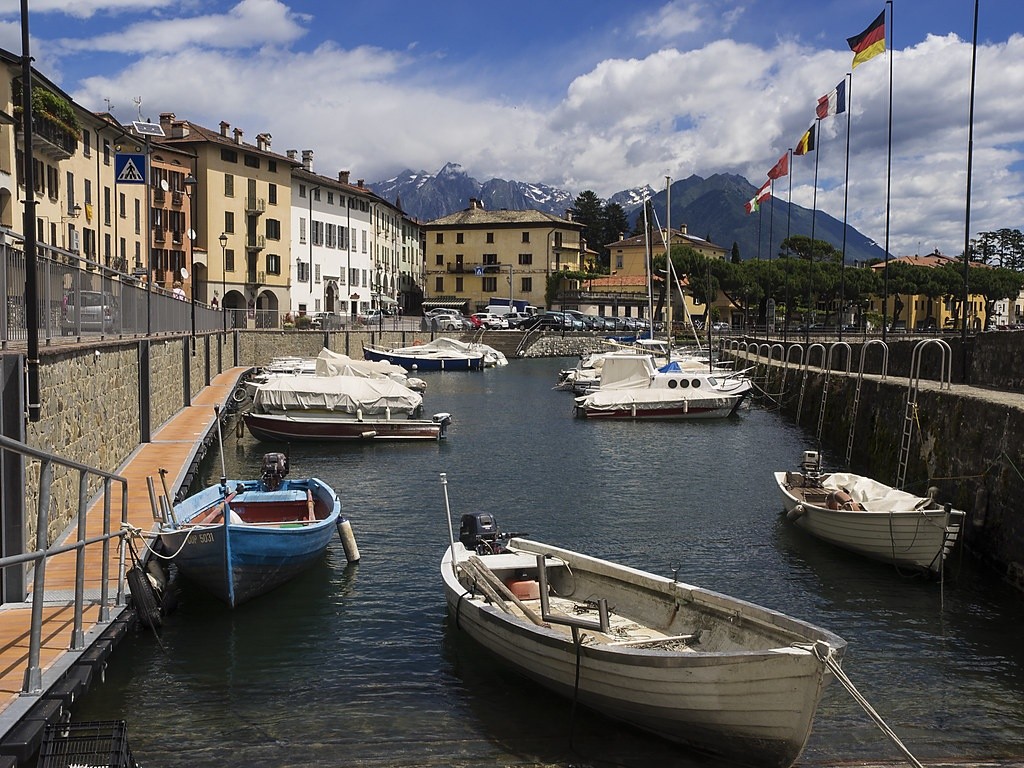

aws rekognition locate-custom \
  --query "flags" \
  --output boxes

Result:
[743,197,759,215]
[766,152,788,179]
[816,78,846,120]
[755,179,770,203]
[846,9,886,70]
[792,124,814,156]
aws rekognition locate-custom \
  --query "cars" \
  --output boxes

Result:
[713,322,732,331]
[418,314,463,331]
[503,312,531,330]
[547,310,690,332]
[498,316,509,330]
[516,314,564,332]
[437,309,464,317]
[359,308,384,325]
[464,315,483,330]
[425,308,445,317]
[455,316,475,330]
[311,312,335,330]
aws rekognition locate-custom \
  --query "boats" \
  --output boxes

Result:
[572,353,758,419]
[242,346,427,420]
[241,411,453,445]
[157,452,340,607]
[437,472,850,768]
[772,449,954,562]
[359,338,509,373]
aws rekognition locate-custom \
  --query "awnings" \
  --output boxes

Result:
[378,296,398,305]
[421,298,469,307]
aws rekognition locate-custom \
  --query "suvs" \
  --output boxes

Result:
[471,313,500,331]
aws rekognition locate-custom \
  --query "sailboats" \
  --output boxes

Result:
[551,175,754,413]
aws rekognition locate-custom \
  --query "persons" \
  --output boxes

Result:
[171,281,187,302]
[212,289,219,310]
[137,274,147,289]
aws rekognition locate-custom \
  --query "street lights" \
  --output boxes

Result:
[218,231,229,331]
[376,265,383,331]
